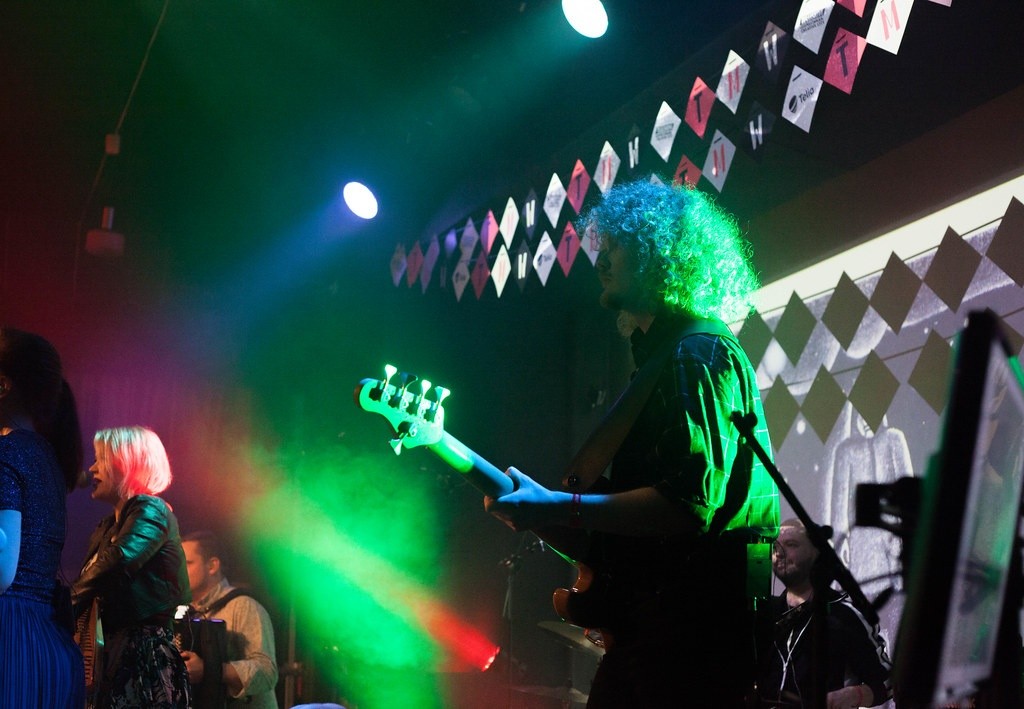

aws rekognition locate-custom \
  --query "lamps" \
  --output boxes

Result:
[84,204,125,259]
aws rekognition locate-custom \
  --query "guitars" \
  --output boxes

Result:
[352,361,611,654]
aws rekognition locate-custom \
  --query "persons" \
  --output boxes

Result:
[0,325,89,709]
[173,532,280,709]
[485,173,782,709]
[67,424,197,709]
[770,514,892,709]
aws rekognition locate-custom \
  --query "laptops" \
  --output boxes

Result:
[890,309,1024,709]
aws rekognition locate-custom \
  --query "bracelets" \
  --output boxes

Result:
[854,685,862,709]
[573,491,581,517]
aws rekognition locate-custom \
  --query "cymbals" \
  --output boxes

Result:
[537,622,606,656]
[502,686,589,703]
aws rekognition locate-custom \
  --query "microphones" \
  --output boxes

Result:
[76,471,91,488]
